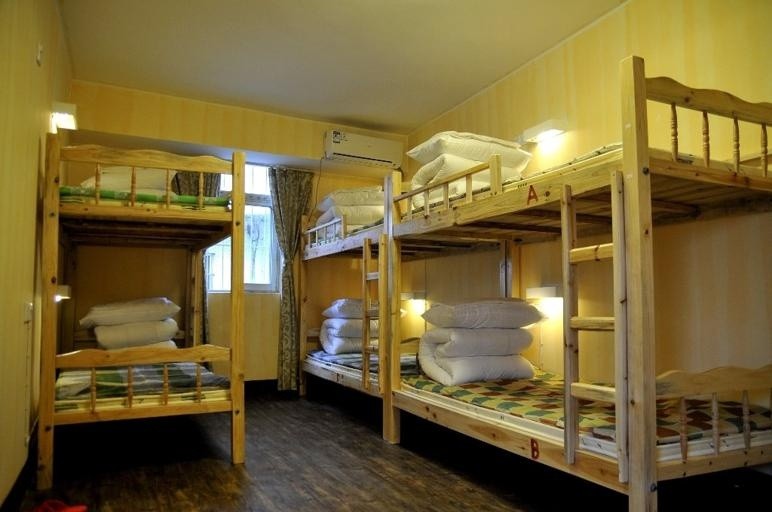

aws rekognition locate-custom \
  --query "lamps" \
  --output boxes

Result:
[51,102,80,133]
[56,285,72,301]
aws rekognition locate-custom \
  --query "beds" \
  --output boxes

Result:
[32,128,249,491]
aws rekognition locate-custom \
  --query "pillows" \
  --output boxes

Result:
[76,296,182,327]
